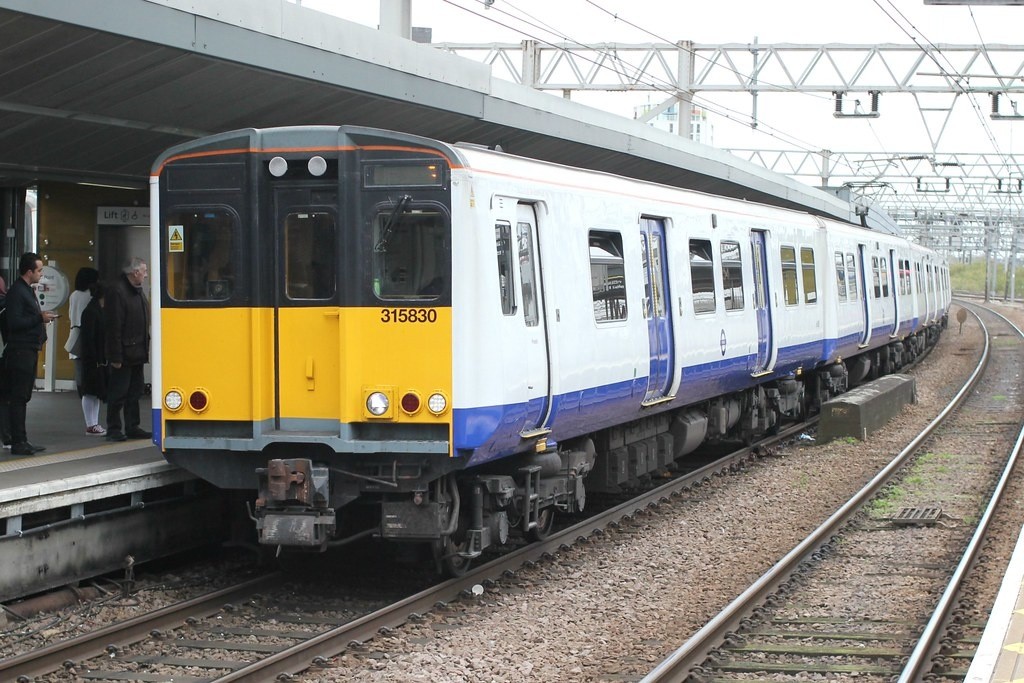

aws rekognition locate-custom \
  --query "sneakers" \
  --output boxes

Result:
[106,432,127,441]
[86,425,106,436]
[96,424,107,434]
[126,427,153,439]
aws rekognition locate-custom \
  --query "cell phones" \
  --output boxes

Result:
[54,314,62,318]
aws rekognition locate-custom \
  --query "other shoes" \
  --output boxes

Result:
[3,443,12,449]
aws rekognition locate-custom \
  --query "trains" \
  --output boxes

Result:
[150,124,952,579]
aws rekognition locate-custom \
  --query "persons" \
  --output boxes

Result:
[105,257,153,441]
[68,267,99,401]
[6,253,54,457]
[0,290,14,450]
[79,282,107,436]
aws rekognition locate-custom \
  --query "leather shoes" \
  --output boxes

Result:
[25,442,46,452]
[11,443,36,456]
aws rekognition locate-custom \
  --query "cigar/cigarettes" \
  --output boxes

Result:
[50,318,52,325]
